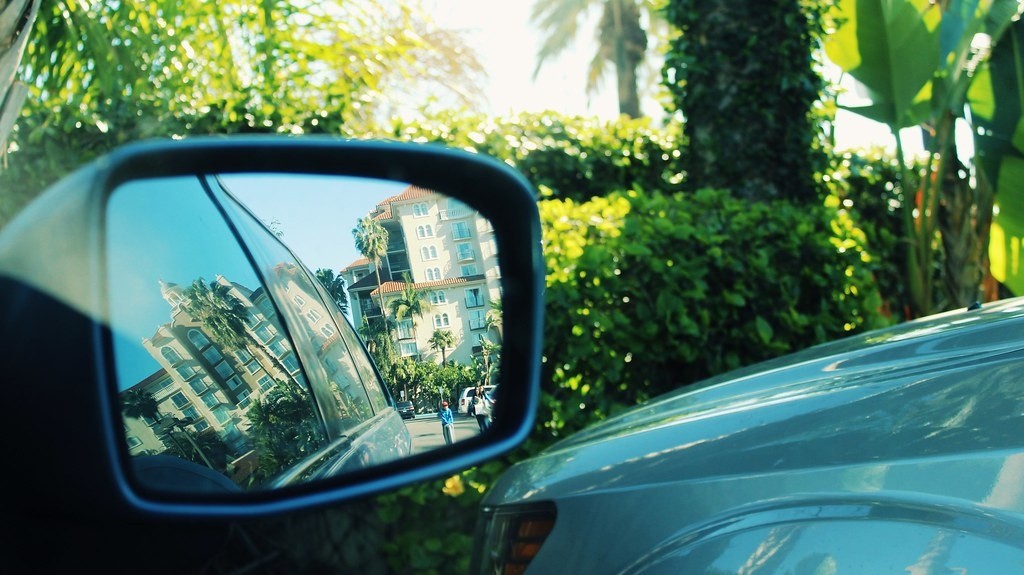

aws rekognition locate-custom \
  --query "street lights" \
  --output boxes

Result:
[157,417,216,471]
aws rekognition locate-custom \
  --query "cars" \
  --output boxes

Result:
[105,173,410,491]
[397,400,416,419]
[466,296,1023,575]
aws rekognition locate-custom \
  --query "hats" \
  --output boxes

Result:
[442,402,448,406]
[474,387,479,390]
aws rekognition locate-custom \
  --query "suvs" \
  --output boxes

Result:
[457,386,498,415]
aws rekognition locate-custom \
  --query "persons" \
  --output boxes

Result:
[440,402,454,445]
[473,380,495,434]
